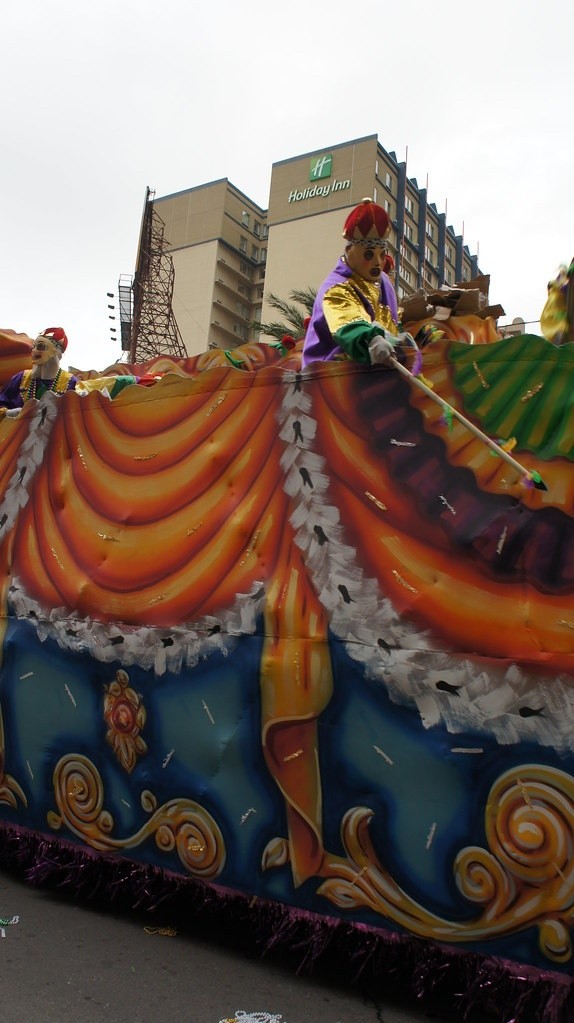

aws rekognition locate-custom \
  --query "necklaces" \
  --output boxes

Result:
[25,365,62,399]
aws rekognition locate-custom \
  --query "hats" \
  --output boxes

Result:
[342,197,390,246]
[39,327,68,353]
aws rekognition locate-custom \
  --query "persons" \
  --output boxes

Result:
[301,239,419,371]
[0,328,78,409]
